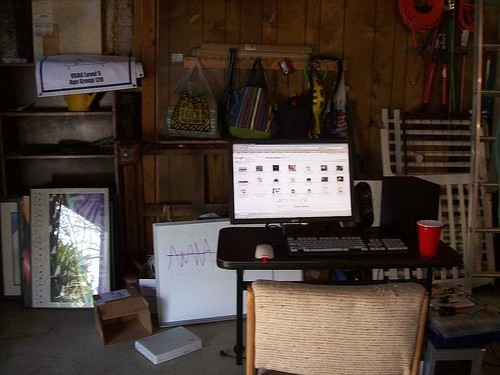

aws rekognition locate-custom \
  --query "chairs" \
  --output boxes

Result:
[245,277,430,375]
[379,112,497,285]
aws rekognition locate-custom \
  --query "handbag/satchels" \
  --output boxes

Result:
[280,94,310,138]
[217,56,280,138]
[321,58,350,139]
[163,56,221,140]
[300,56,327,137]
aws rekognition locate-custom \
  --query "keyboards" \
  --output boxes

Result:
[282,238,409,255]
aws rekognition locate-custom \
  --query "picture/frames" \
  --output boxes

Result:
[0,199,23,296]
[30,186,113,309]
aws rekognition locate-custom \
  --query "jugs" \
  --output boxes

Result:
[64,93,98,111]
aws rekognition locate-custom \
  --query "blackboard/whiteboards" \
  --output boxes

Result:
[153,219,307,330]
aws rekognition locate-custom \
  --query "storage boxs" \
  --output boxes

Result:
[134,325,203,365]
[93,285,153,345]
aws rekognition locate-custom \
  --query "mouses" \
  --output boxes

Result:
[255,244,274,262]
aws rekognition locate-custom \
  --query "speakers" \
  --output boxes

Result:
[353,182,374,235]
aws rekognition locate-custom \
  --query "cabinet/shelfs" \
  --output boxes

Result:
[0,63,122,294]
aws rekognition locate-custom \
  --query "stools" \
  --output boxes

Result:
[426,341,486,375]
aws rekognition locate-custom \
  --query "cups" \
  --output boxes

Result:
[416,219,443,257]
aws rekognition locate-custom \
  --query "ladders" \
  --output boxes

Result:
[465,0,499,303]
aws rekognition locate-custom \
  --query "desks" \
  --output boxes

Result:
[216,221,463,367]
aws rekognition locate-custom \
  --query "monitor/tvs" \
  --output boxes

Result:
[228,136,356,227]
